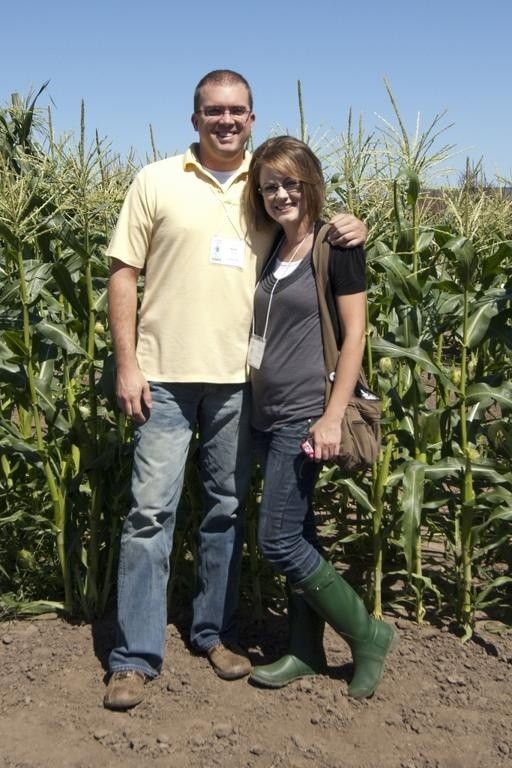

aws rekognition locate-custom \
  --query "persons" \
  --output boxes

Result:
[248,134,398,700]
[101,72,371,710]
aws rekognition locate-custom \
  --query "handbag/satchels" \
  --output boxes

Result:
[325,351,384,479]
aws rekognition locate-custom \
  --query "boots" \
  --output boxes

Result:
[248,577,327,689]
[287,554,394,700]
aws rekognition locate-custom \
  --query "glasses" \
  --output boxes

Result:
[195,106,252,119]
[257,178,301,194]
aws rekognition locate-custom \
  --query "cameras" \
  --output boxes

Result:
[300,437,314,460]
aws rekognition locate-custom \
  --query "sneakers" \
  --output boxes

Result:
[103,669,146,708]
[206,641,251,680]
